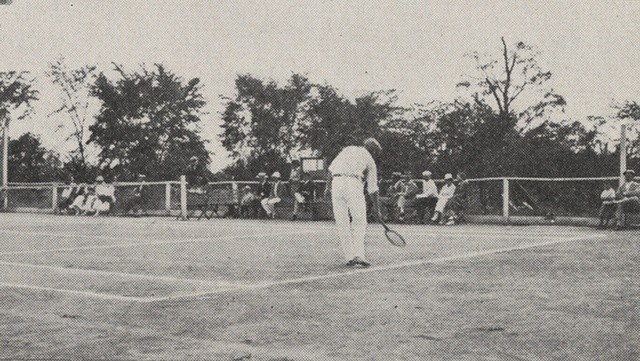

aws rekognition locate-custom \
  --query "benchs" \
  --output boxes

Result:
[209,189,242,216]
[116,189,151,218]
[243,188,321,218]
[173,188,207,219]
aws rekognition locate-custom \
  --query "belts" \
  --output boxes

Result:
[334,174,359,180]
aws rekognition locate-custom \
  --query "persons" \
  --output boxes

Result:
[324,138,383,267]
[123,175,152,216]
[258,170,269,198]
[243,186,254,216]
[431,175,457,222]
[616,170,640,229]
[260,172,283,217]
[52,170,116,216]
[413,170,439,223]
[599,180,618,229]
[186,157,210,195]
[288,161,306,195]
[389,170,419,223]
[447,173,467,225]
[292,172,316,219]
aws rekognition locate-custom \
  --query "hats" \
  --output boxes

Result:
[95,176,104,182]
[255,173,269,179]
[444,173,454,183]
[270,172,281,179]
[422,171,432,177]
[623,170,636,177]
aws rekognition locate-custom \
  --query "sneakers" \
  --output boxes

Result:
[438,219,446,225]
[346,260,353,266]
[448,216,455,220]
[431,213,439,222]
[446,221,455,226]
[353,256,372,266]
